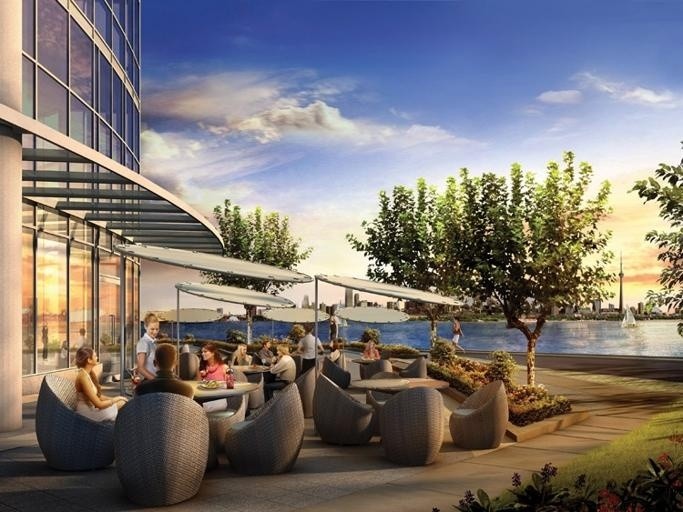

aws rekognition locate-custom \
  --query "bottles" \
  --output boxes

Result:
[224,361,234,388]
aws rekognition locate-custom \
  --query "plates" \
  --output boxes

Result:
[200,383,222,389]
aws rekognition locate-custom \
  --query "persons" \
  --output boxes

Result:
[157,332,172,344]
[74,346,129,422]
[227,342,251,365]
[77,329,86,349]
[297,323,324,374]
[194,341,234,412]
[134,312,159,380]
[178,332,196,355]
[262,343,297,404]
[132,344,194,398]
[60,340,75,364]
[40,321,49,362]
[319,338,344,370]
[451,315,465,355]
[256,339,275,365]
[328,309,338,346]
[359,338,381,361]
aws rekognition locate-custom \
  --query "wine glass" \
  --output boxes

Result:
[260,358,266,368]
